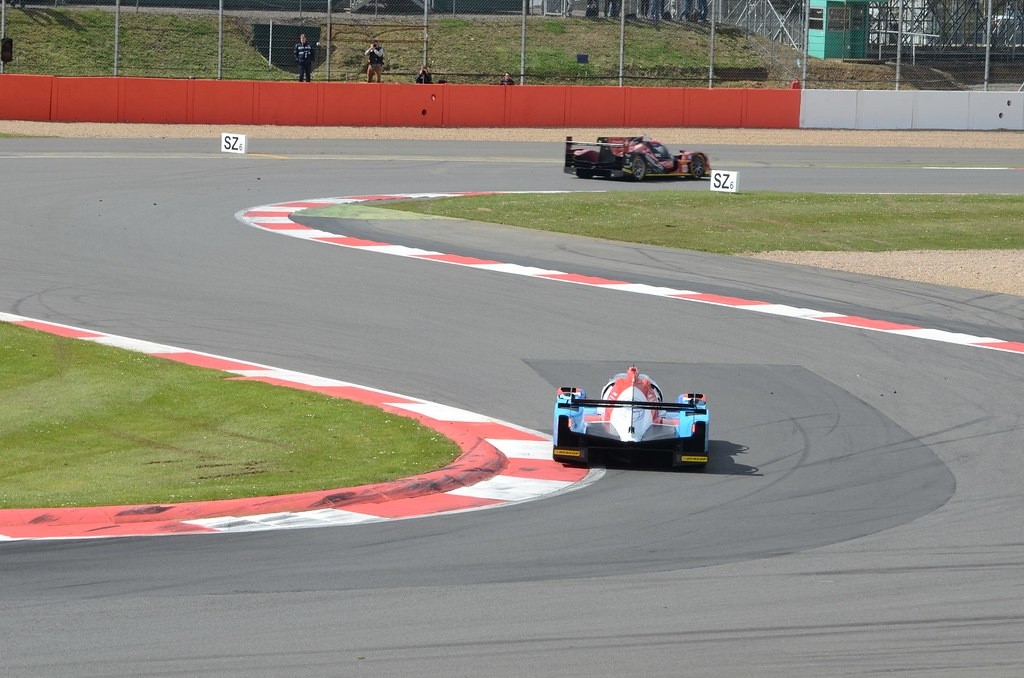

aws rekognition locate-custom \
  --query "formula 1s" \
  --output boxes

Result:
[549,364,712,468]
[562,135,714,182]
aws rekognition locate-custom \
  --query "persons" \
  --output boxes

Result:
[416,66,431,84]
[365,40,384,83]
[500,72,514,85]
[564,0,622,19]
[680,0,708,22]
[294,33,315,82]
[641,0,671,22]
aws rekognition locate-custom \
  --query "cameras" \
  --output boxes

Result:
[373,45,376,47]
[422,70,426,75]
